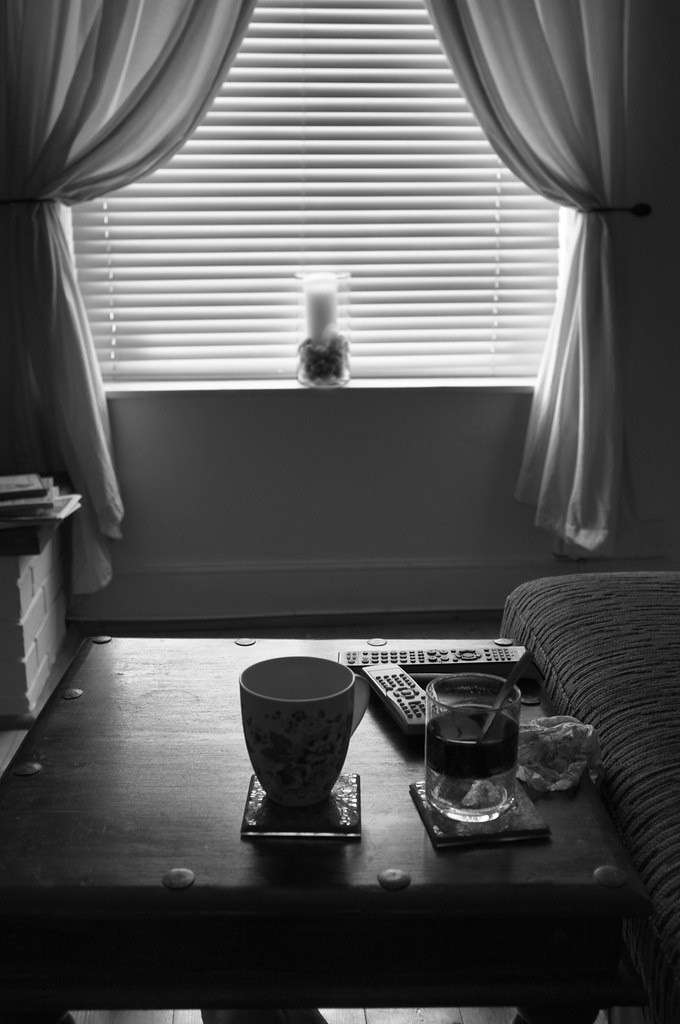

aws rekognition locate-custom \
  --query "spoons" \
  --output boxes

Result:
[436,650,534,809]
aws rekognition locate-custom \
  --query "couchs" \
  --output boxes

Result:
[501,572,680,1024]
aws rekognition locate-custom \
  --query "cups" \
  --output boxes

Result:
[425,674,520,822]
[241,656,370,806]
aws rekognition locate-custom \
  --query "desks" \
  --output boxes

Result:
[0,636,648,1024]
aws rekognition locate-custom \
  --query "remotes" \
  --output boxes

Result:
[338,646,545,682]
[360,663,428,736]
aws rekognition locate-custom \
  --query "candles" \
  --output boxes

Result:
[303,271,339,348]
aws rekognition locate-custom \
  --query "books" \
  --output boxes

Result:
[0,473,48,501]
[0,478,83,529]
